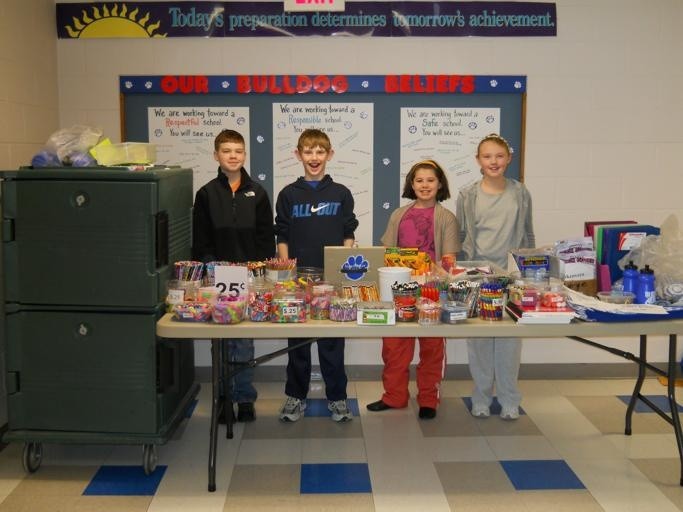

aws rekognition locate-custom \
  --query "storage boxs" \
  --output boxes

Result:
[435,259,506,285]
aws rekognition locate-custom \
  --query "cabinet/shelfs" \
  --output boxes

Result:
[0,167,200,477]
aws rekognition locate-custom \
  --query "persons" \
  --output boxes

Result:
[366,160,461,420]
[273,127,360,422]
[192,129,275,422]
[455,133,537,422]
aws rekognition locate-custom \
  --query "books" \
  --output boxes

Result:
[584,220,660,284]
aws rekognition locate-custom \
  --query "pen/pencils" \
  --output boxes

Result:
[417,283,439,326]
[428,278,448,306]
[264,256,297,271]
[204,260,247,287]
[343,285,379,303]
[247,261,264,278]
[391,281,418,321]
[448,281,480,318]
[174,261,204,281]
[480,282,504,321]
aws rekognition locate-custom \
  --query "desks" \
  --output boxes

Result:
[156,310,683,492]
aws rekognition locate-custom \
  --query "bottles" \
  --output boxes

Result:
[622,261,639,304]
[166,255,567,326]
[637,264,656,305]
